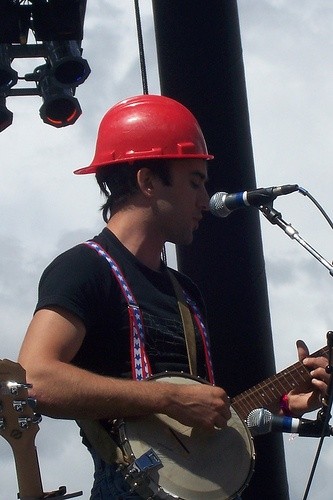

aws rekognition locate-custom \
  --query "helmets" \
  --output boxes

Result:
[74,94,216,176]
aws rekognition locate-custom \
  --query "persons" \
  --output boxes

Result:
[17,96,331,500]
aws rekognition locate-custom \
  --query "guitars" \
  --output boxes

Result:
[0,359,83,500]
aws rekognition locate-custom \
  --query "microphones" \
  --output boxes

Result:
[246,407,333,437]
[208,184,299,218]
